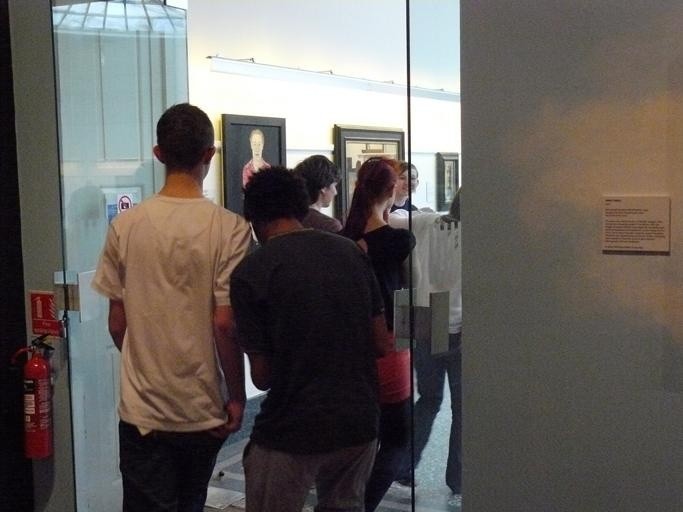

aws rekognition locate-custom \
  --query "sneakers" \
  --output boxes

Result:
[448,493,462,507]
[384,481,412,503]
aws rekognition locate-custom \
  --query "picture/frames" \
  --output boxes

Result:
[222,113,286,216]
[437,152,458,212]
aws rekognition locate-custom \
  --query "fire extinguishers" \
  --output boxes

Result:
[12,331,55,460]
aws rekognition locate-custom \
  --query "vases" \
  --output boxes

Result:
[334,124,405,226]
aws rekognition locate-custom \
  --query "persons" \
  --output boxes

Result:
[242,129,270,190]
[91,101,255,511]
[332,155,418,512]
[387,161,417,211]
[389,185,461,498]
[227,165,391,512]
[292,154,344,234]
